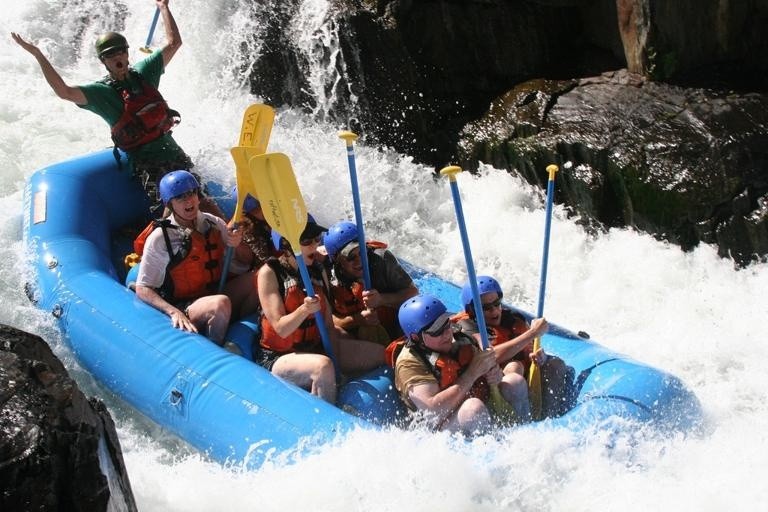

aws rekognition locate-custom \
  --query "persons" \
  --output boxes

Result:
[9,0,207,208]
[393,272,545,438]
[136,167,297,350]
[255,210,420,406]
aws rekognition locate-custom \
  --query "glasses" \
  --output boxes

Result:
[102,50,127,61]
[470,294,504,313]
[424,311,451,338]
[298,236,323,248]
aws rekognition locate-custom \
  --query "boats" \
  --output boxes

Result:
[25,144,707,475]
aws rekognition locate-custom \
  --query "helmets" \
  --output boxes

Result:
[230,183,259,216]
[462,275,505,314]
[322,220,364,265]
[394,294,449,344]
[157,169,200,203]
[94,32,130,60]
[270,212,329,252]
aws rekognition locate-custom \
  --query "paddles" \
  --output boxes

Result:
[528,164,559,422]
[440,166,517,424]
[249,153,339,379]
[220,104,276,293]
[231,147,266,201]
